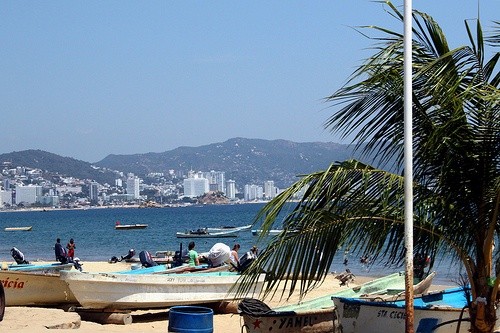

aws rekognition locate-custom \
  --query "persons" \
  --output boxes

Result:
[230,244,258,263]
[188,241,199,265]
[55,238,76,260]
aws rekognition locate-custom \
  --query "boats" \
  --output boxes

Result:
[250,229,296,237]
[0,243,269,311]
[330,280,500,332]
[4,226,33,232]
[114,221,149,231]
[175,224,252,239]
[235,249,436,333]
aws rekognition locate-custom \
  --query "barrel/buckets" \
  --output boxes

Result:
[168,306,213,333]
